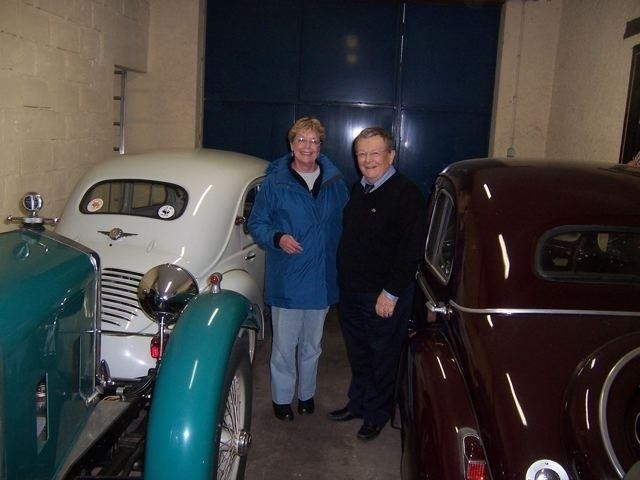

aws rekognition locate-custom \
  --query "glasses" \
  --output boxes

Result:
[358,151,380,157]
[296,138,318,145]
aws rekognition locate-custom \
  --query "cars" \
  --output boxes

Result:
[400,157,640,480]
[0,191,263,480]
[0,149,272,399]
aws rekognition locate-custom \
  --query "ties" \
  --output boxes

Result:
[363,183,375,195]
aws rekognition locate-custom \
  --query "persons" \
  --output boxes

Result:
[327,127,427,441]
[246,115,351,421]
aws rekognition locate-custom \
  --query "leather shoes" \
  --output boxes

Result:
[272,400,294,422]
[356,411,391,441]
[297,397,315,416]
[326,405,359,422]
